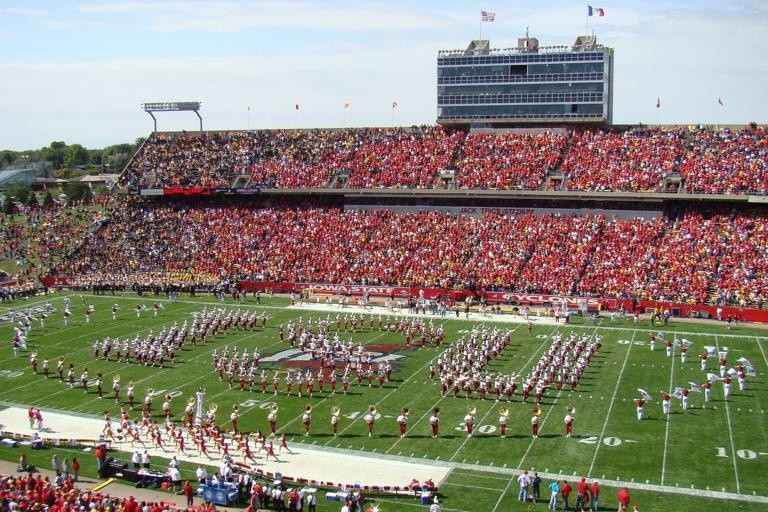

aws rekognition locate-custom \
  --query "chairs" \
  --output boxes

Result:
[235,460,439,500]
[47,125,768,305]
[0,473,225,512]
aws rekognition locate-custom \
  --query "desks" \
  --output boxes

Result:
[106,459,170,487]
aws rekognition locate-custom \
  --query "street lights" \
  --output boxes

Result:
[21,155,29,185]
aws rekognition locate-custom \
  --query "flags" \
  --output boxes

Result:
[481,10,496,22]
[589,6,604,16]
[656,98,660,108]
[392,96,398,109]
[719,98,723,106]
[296,104,299,109]
[344,99,350,110]
